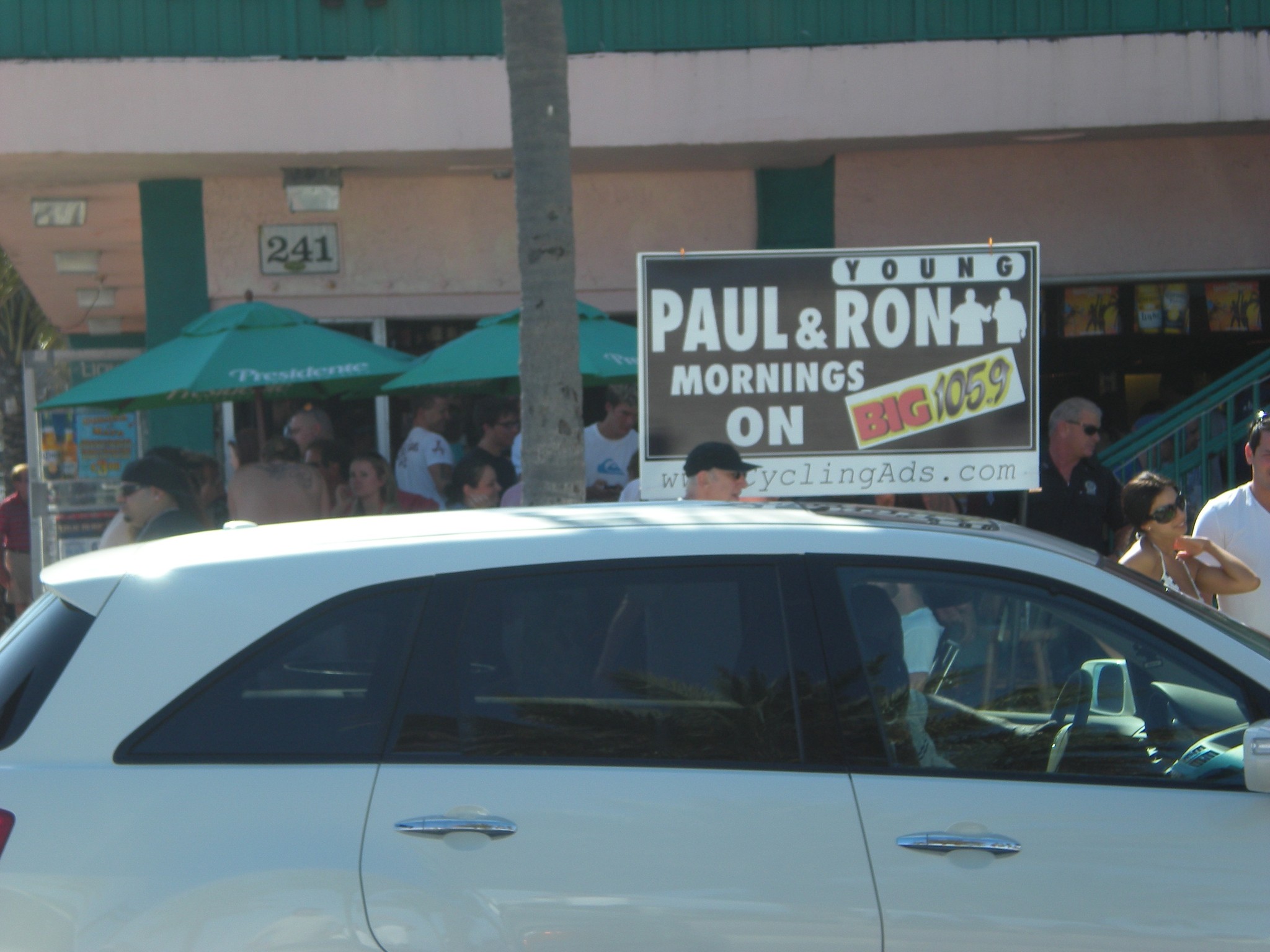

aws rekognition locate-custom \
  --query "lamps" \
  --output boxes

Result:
[74,286,118,308]
[88,316,122,338]
[29,196,89,228]
[55,248,105,274]
[283,166,343,214]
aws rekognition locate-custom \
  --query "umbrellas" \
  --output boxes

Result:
[380,296,641,401]
[34,294,416,460]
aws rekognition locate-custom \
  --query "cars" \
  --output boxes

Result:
[0,492,1270,951]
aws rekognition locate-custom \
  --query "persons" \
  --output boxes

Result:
[1084,472,1262,669]
[105,383,643,539]
[2,464,30,624]
[1188,411,1270,637]
[998,400,1127,562]
[1109,403,1178,490]
[594,439,763,695]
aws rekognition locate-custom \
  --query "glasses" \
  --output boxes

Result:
[1064,419,1103,437]
[495,419,520,429]
[730,471,747,479]
[1249,411,1270,430]
[121,480,151,496]
[1148,491,1187,524]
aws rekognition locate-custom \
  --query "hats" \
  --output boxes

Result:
[121,447,201,507]
[683,442,762,477]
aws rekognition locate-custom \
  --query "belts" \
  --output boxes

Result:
[12,549,29,553]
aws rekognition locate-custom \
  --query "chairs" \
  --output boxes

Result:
[846,578,923,772]
